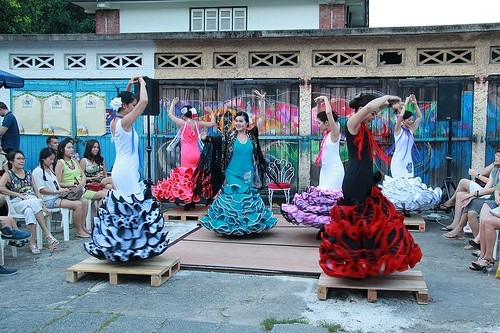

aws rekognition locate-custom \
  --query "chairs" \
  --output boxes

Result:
[51,208,71,243]
[4,192,52,250]
[266,159,294,209]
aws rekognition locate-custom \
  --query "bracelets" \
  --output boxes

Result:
[477,190,479,197]
[476,173,480,179]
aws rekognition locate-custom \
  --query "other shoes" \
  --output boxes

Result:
[8,239,28,246]
[472,250,481,257]
[469,239,480,250]
[469,257,486,271]
[185,202,196,210]
[471,258,495,268]
[464,245,473,250]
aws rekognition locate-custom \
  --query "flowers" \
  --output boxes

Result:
[189,107,197,114]
[110,97,122,111]
[181,107,187,114]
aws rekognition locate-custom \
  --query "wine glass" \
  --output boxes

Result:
[486,264,493,277]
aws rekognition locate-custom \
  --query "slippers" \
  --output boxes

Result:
[441,232,465,240]
[439,203,455,210]
[442,226,453,231]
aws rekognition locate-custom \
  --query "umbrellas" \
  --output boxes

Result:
[0,70,24,89]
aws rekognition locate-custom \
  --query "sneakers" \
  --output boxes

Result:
[0,265,18,276]
[1,229,32,240]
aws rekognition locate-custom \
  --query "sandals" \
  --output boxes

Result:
[44,233,59,247]
[29,245,41,254]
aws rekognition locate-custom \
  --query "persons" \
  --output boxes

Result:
[84,75,169,262]
[279,95,345,240]
[377,94,443,217]
[438,148,500,273]
[0,102,113,277]
[318,90,423,281]
[150,97,216,210]
[198,89,278,236]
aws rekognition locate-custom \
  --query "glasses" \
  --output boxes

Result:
[14,157,26,161]
[494,154,500,158]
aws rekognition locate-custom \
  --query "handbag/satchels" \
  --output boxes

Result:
[84,179,104,190]
[63,187,83,200]
